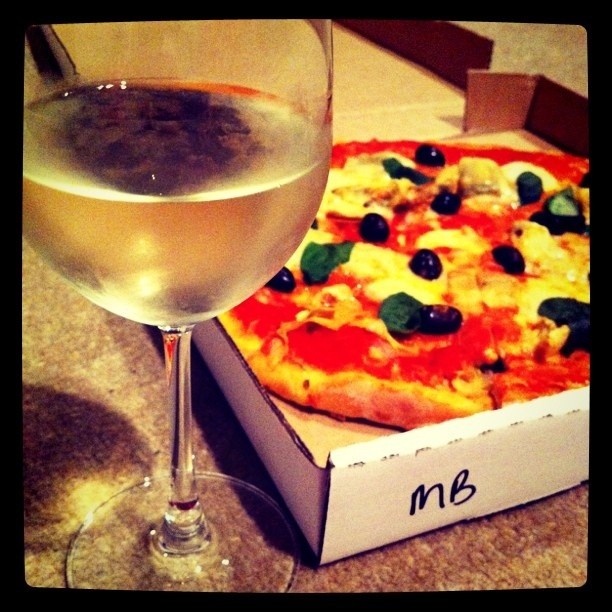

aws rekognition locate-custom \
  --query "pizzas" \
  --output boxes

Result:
[218,140,588,428]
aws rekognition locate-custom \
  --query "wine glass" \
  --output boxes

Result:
[21,19,335,593]
